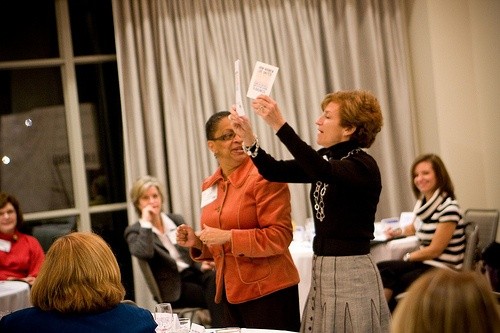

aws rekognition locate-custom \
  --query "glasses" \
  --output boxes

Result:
[211,132,236,140]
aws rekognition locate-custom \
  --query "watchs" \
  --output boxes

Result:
[403,252,410,263]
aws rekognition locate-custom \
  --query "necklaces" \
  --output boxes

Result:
[312,147,361,222]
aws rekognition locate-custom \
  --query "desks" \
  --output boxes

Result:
[0,281,33,318]
[288,223,419,321]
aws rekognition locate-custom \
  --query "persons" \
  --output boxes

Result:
[123,176,231,328]
[376,153,466,309]
[229,89,391,333]
[392,269,500,333]
[0,232,158,333]
[474,241,500,291]
[0,193,45,285]
[176,111,302,332]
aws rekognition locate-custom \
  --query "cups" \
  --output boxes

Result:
[174,318,191,333]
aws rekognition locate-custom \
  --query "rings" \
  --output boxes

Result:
[259,106,264,110]
[227,115,231,120]
[202,239,206,244]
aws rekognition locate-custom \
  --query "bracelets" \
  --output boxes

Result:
[240,135,259,159]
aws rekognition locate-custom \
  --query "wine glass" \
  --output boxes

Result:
[155,303,173,333]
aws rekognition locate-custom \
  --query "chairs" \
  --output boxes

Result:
[139,260,201,320]
[462,209,499,252]
[396,222,479,301]
